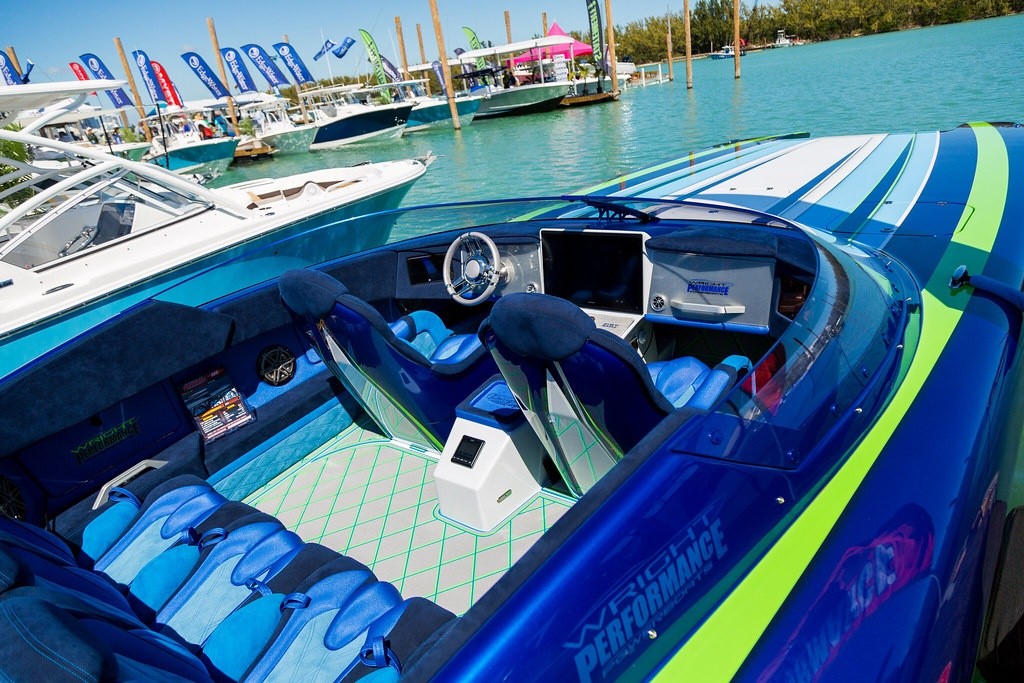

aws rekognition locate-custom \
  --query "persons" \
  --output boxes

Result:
[211,109,280,127]
[222,131,229,137]
[198,123,213,140]
[138,123,147,141]
[503,74,510,88]
[53,135,59,140]
[178,122,185,132]
[59,131,74,142]
[113,127,121,143]
[84,126,98,144]
[508,72,516,86]
[70,132,83,141]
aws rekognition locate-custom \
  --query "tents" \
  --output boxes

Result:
[506,18,592,77]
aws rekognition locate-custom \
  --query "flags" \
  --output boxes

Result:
[332,36,356,58]
[313,40,336,61]
[20,59,34,81]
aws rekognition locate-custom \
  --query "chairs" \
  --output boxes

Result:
[280,268,498,452]
[0,473,460,683]
[480,294,757,498]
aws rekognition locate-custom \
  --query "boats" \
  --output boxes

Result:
[774,31,793,48]
[569,76,631,97]
[11,98,151,184]
[708,44,744,60]
[450,35,576,120]
[155,92,320,155]
[0,119,1024,683]
[297,65,486,134]
[98,108,240,183]
[0,79,439,385]
[277,29,418,154]
